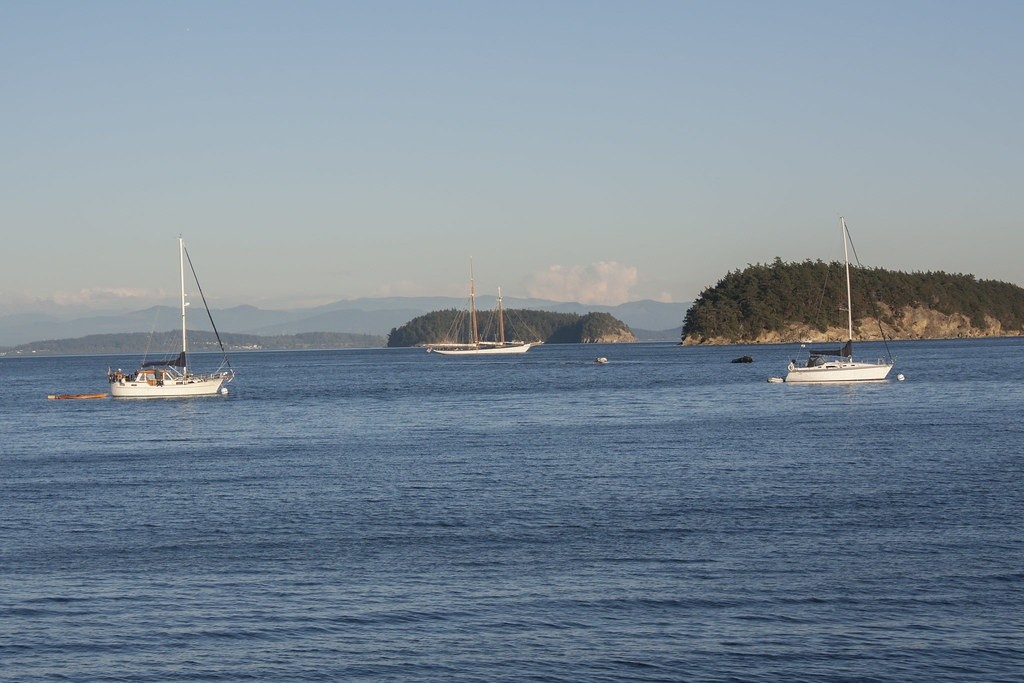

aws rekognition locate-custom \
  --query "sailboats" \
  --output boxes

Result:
[783,214,896,382]
[421,257,546,356]
[105,231,236,397]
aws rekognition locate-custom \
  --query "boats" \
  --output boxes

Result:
[47,392,109,400]
